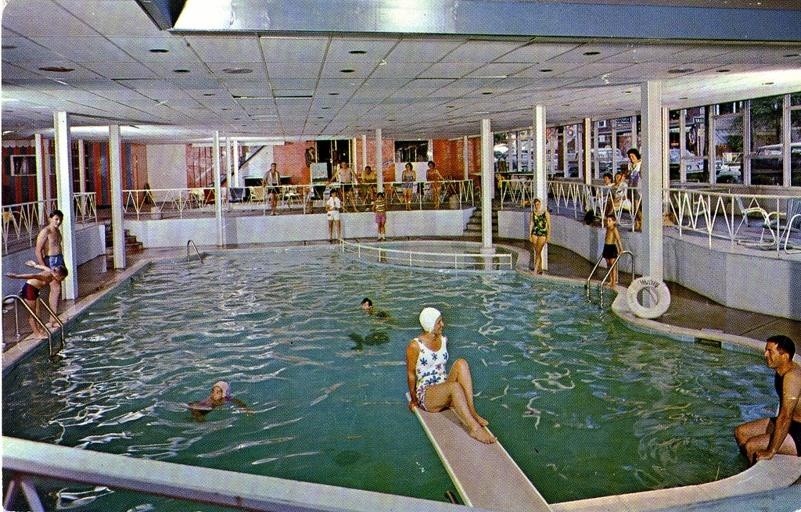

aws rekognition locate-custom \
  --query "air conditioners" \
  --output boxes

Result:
[597,120,607,129]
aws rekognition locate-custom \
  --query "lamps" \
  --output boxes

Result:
[189,142,294,146]
[494,143,509,161]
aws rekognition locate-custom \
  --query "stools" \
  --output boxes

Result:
[151,207,161,220]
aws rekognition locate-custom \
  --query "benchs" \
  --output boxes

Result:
[590,179,636,228]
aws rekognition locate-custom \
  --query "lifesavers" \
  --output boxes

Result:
[626,277,671,318]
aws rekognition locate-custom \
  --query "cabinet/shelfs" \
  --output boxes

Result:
[471,172,481,191]
[74,155,91,182]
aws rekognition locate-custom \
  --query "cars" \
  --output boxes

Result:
[498,145,635,179]
[669,149,722,183]
[715,142,800,188]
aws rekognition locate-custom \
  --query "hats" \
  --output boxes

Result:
[211,380,230,398]
[419,307,441,332]
[533,197,545,210]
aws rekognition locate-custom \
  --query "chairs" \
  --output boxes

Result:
[189,187,226,208]
[670,184,801,254]
[494,173,615,200]
[341,182,456,206]
[245,185,302,207]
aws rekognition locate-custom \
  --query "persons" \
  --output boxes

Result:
[361,298,392,320]
[4,260,68,340]
[325,161,387,241]
[183,381,254,424]
[426,161,444,209]
[402,163,416,210]
[35,210,65,328]
[529,199,550,275]
[262,163,281,215]
[406,307,497,445]
[601,149,642,288]
[734,335,801,464]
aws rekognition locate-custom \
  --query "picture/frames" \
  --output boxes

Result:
[9,154,55,176]
